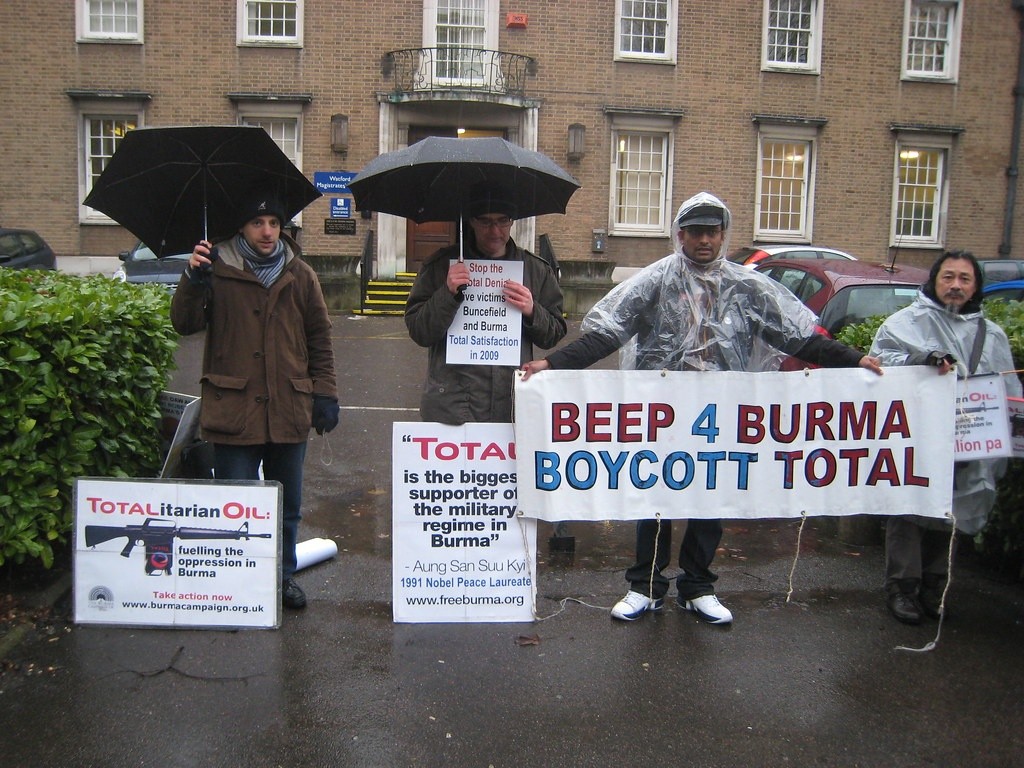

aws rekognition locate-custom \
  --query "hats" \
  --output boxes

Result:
[679,203,729,231]
[463,190,518,217]
[239,189,285,229]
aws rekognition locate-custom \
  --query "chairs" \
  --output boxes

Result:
[790,279,813,300]
[824,295,914,329]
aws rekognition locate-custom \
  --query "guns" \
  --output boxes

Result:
[83,517,272,577]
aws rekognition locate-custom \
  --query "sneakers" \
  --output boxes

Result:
[611,590,665,621]
[676,594,733,624]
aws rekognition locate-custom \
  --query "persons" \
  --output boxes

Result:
[521,191,883,623]
[405,181,568,426]
[868,250,1024,623]
[170,192,339,608]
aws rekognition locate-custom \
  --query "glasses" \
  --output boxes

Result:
[474,216,513,227]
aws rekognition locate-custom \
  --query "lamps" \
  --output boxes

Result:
[566,122,586,161]
[330,114,349,154]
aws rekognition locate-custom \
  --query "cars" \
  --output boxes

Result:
[976,258,1024,304]
[748,258,931,373]
[726,242,859,305]
[0,226,57,272]
[111,238,193,296]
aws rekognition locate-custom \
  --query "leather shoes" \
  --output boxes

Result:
[917,585,949,619]
[887,595,923,624]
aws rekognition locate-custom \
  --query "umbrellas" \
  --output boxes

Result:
[83,125,324,268]
[345,135,581,292]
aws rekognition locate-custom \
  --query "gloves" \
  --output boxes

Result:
[191,246,217,286]
[312,396,340,435]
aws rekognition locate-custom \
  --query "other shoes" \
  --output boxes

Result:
[282,580,306,607]
